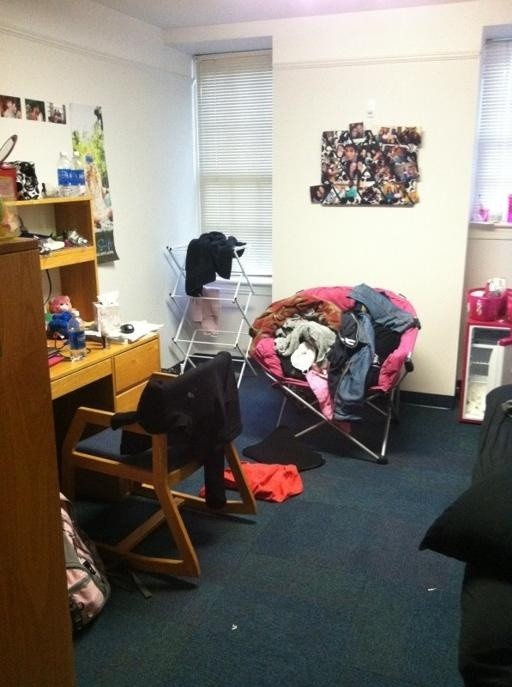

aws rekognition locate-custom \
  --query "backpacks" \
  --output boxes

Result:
[59,492,112,634]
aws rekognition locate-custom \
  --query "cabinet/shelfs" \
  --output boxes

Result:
[0,195,160,412]
[1,239,77,686]
[460,315,512,424]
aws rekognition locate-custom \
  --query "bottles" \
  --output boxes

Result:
[57,151,72,198]
[70,151,86,198]
[66,310,86,362]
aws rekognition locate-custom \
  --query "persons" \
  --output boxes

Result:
[3,98,21,119]
[311,122,421,207]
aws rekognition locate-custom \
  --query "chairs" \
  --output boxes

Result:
[249,287,417,465]
[59,351,256,578]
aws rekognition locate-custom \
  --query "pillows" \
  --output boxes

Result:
[418,465,512,580]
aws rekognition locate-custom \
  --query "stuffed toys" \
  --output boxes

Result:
[46,294,79,339]
